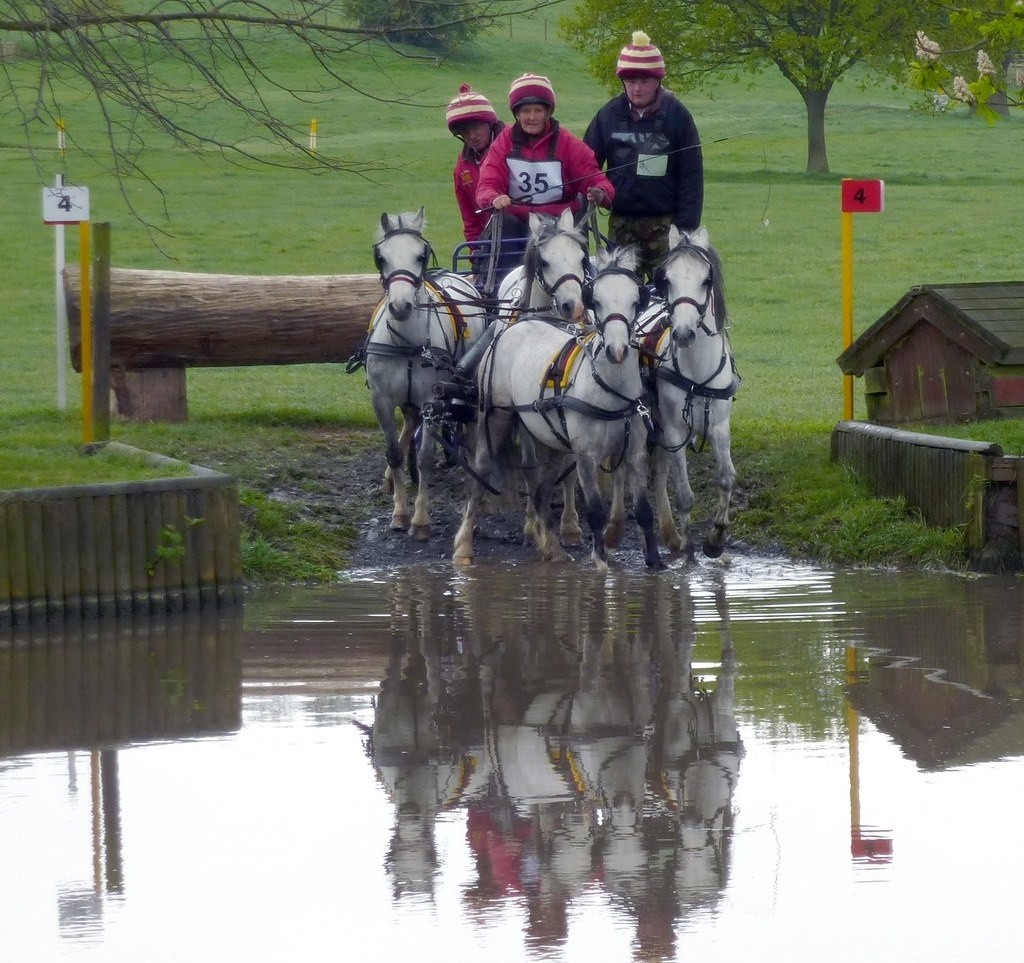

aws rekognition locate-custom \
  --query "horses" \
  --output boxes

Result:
[364,206,739,574]
[350,574,749,963]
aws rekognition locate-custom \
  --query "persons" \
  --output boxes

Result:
[470,73,615,309]
[447,83,509,263]
[583,30,705,285]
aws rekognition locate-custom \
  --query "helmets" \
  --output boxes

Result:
[617,31,666,80]
[509,73,555,116]
[446,84,499,135]
